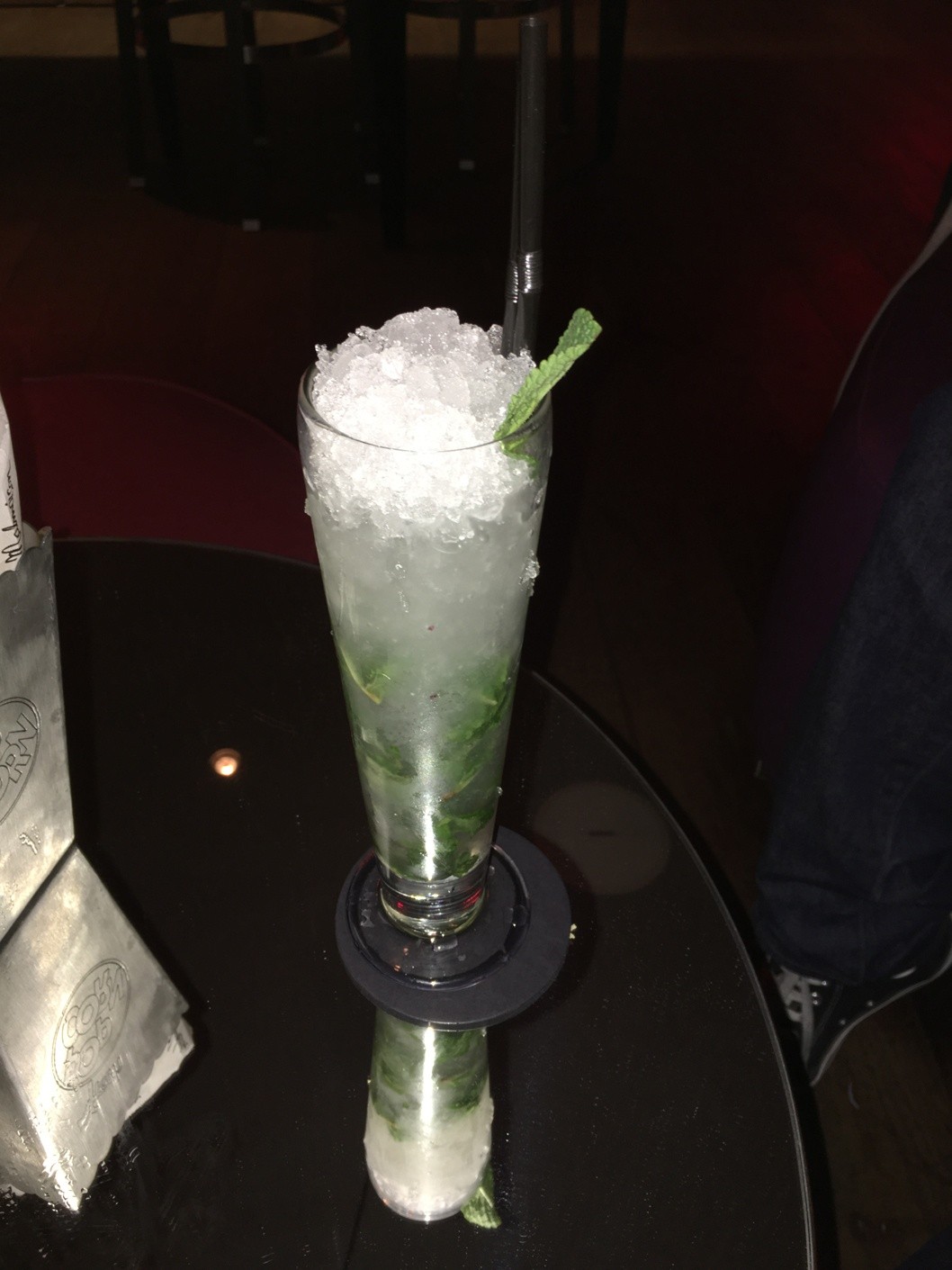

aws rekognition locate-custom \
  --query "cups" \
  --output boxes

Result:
[360,1014,492,1225]
[300,344,555,938]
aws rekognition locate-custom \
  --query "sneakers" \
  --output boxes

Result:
[771,963,840,1068]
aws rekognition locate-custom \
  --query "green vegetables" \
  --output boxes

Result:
[492,309,602,465]
[369,1006,492,1136]
[460,1163,504,1230]
[333,628,519,885]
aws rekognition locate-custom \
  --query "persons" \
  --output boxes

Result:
[737,192,952,1094]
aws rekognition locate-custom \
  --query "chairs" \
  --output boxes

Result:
[0,373,322,570]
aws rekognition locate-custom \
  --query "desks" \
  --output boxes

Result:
[0,539,814,1270]
